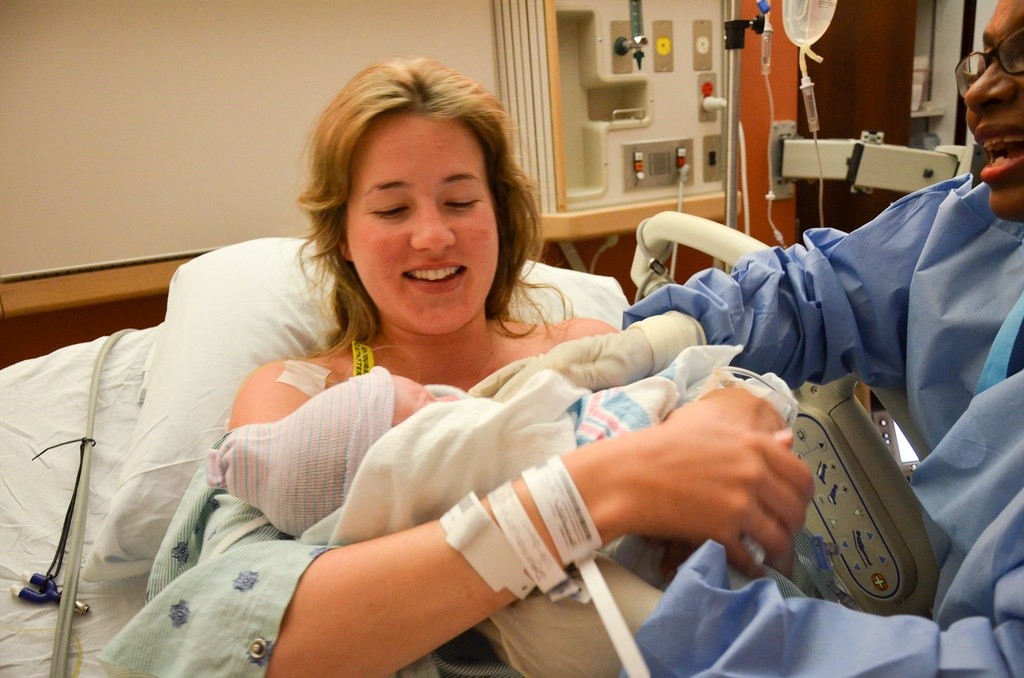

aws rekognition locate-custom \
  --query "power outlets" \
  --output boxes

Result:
[697,72,718,123]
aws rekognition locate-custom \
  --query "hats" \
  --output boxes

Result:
[204,364,396,539]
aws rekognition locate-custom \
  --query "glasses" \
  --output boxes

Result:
[954,28,1024,98]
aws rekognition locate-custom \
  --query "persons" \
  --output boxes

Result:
[208,343,802,554]
[463,0,1024,678]
[92,55,814,678]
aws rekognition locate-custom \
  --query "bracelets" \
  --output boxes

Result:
[440,454,651,678]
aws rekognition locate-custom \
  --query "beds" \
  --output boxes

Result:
[0,212,916,678]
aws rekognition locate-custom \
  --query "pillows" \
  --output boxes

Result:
[75,235,637,582]
